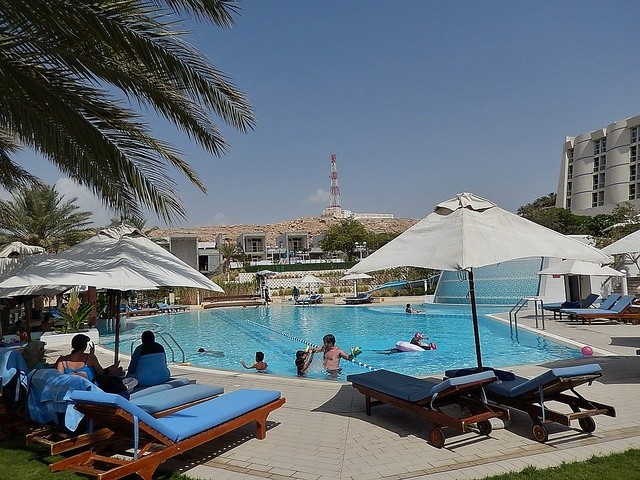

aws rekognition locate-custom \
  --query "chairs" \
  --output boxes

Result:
[538,295,599,319]
[296,295,323,307]
[446,362,616,443]
[48,390,285,480]
[125,304,157,316]
[342,293,372,304]
[346,368,512,448]
[156,303,187,315]
[1,342,28,442]
[566,295,640,325]
[26,375,224,457]
[346,294,363,299]
[560,293,622,322]
[7,368,189,437]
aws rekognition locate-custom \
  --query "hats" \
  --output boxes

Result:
[122,377,138,394]
[72,334,90,349]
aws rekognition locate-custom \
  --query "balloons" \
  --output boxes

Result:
[582,347,593,355]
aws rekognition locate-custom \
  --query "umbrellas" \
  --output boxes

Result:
[294,275,325,298]
[339,274,375,298]
[600,230,640,271]
[536,260,626,309]
[0,221,226,366]
[343,193,616,373]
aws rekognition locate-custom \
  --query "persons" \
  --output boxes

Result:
[296,351,312,359]
[312,334,353,370]
[295,350,314,376]
[406,304,425,313]
[17,328,27,341]
[127,330,170,387]
[57,334,123,392]
[40,317,51,332]
[292,286,300,304]
[410,332,431,350]
[241,352,267,370]
[430,342,437,349]
[21,339,122,377]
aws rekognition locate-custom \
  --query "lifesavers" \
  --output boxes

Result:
[396,342,430,352]
